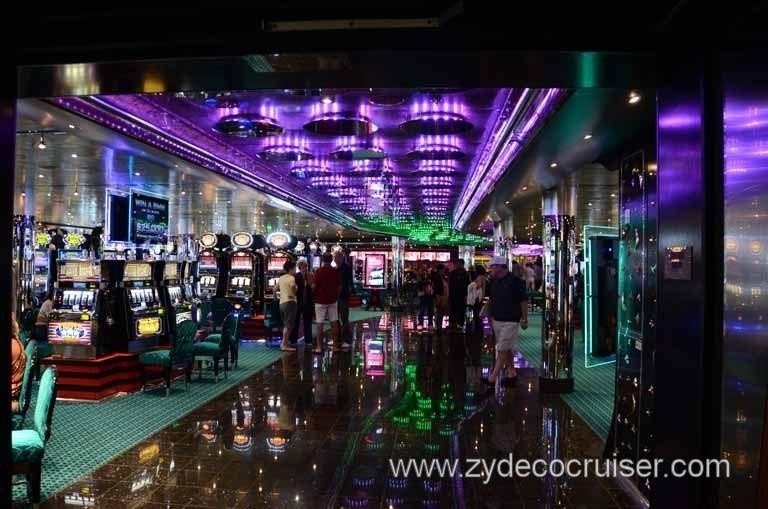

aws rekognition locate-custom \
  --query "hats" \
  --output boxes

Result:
[485,256,507,266]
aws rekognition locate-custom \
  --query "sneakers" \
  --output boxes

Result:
[501,373,517,382]
[417,324,436,332]
[479,377,495,388]
[279,340,351,355]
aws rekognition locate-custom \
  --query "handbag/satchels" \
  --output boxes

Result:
[478,296,493,317]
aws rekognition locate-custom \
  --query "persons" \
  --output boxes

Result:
[24,292,52,340]
[11,312,26,402]
[270,251,542,389]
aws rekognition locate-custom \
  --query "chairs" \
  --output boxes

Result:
[12,333,58,506]
[141,313,242,402]
